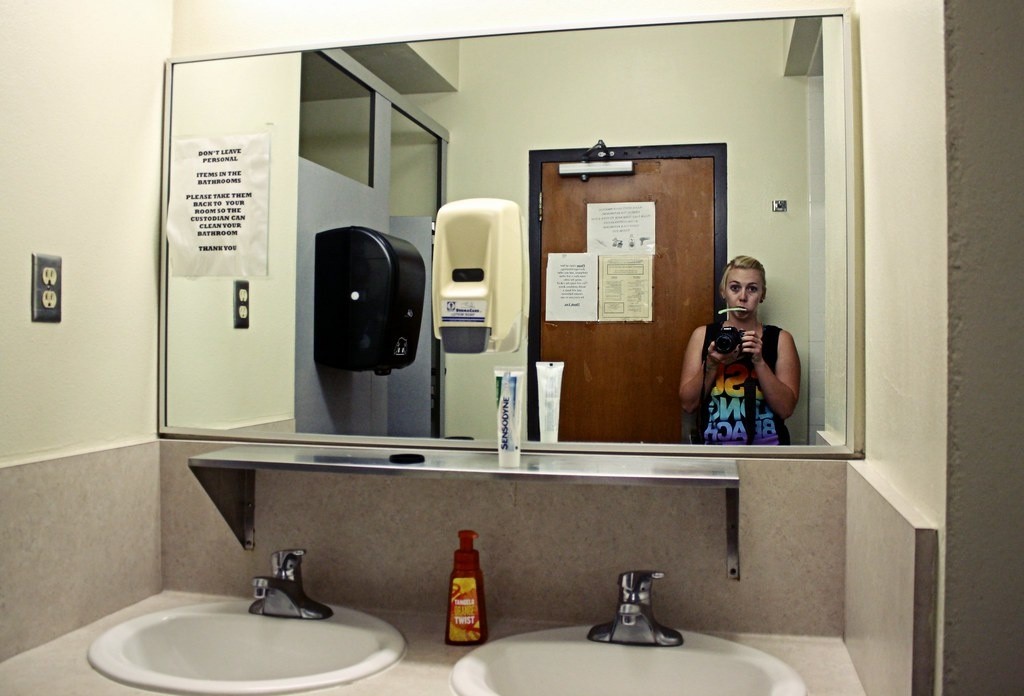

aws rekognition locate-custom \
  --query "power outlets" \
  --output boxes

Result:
[234,280,250,329]
[32,253,62,322]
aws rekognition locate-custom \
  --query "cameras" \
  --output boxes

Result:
[714,326,747,355]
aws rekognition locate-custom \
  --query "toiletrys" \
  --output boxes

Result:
[533,358,566,444]
[492,363,528,472]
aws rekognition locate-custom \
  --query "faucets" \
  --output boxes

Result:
[245,546,335,621]
[585,567,686,649]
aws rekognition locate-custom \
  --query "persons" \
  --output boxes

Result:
[678,256,801,445]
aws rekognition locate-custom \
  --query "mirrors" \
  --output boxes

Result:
[153,4,855,453]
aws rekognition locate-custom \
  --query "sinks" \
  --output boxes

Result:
[84,596,408,696]
[451,618,808,696]
